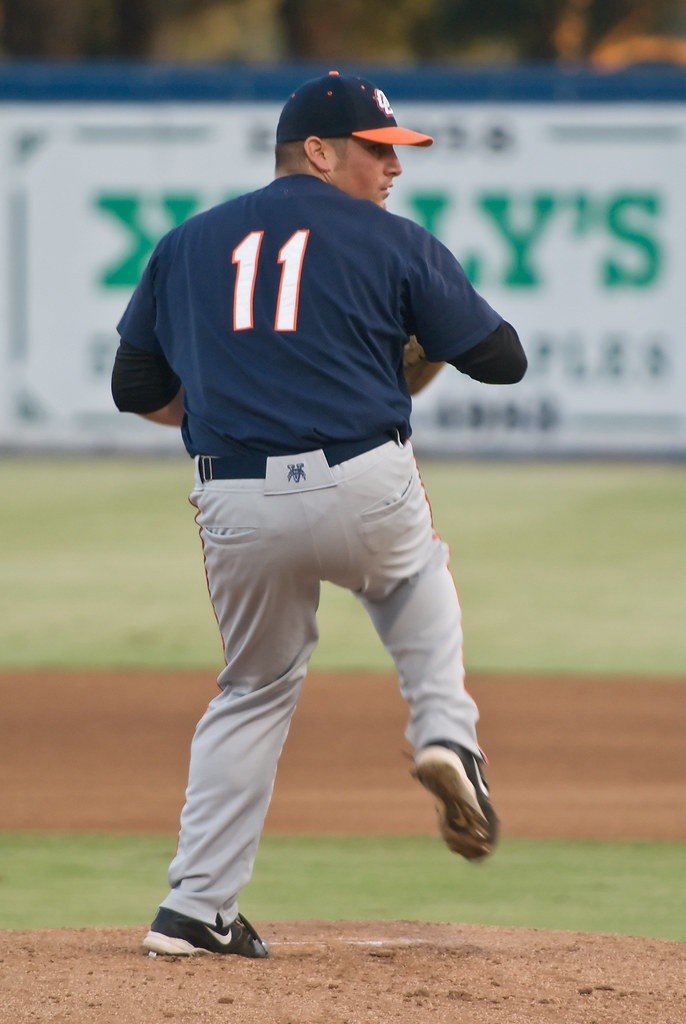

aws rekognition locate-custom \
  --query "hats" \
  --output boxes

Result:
[276,70,433,147]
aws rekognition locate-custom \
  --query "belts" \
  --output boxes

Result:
[199,430,395,482]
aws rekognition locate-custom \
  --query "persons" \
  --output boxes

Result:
[111,72,530,957]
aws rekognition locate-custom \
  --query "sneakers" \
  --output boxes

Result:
[142,909,269,958]
[416,739,501,864]
